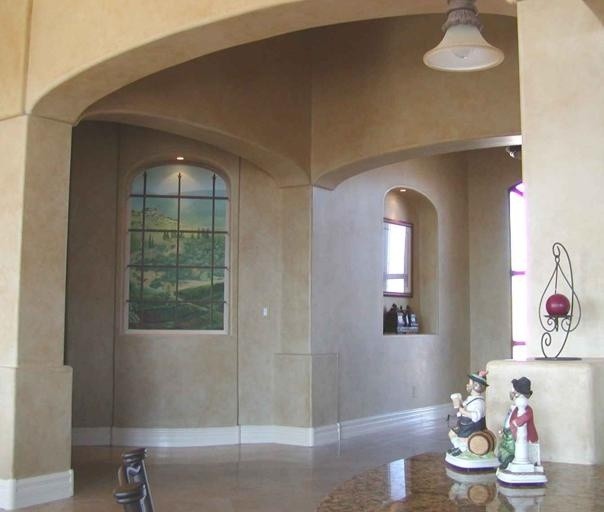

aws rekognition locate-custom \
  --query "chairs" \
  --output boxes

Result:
[114,447,153,512]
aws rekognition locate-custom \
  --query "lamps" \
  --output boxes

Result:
[422,0,505,70]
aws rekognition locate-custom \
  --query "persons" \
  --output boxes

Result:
[443,367,490,457]
[388,302,414,328]
[494,373,540,468]
